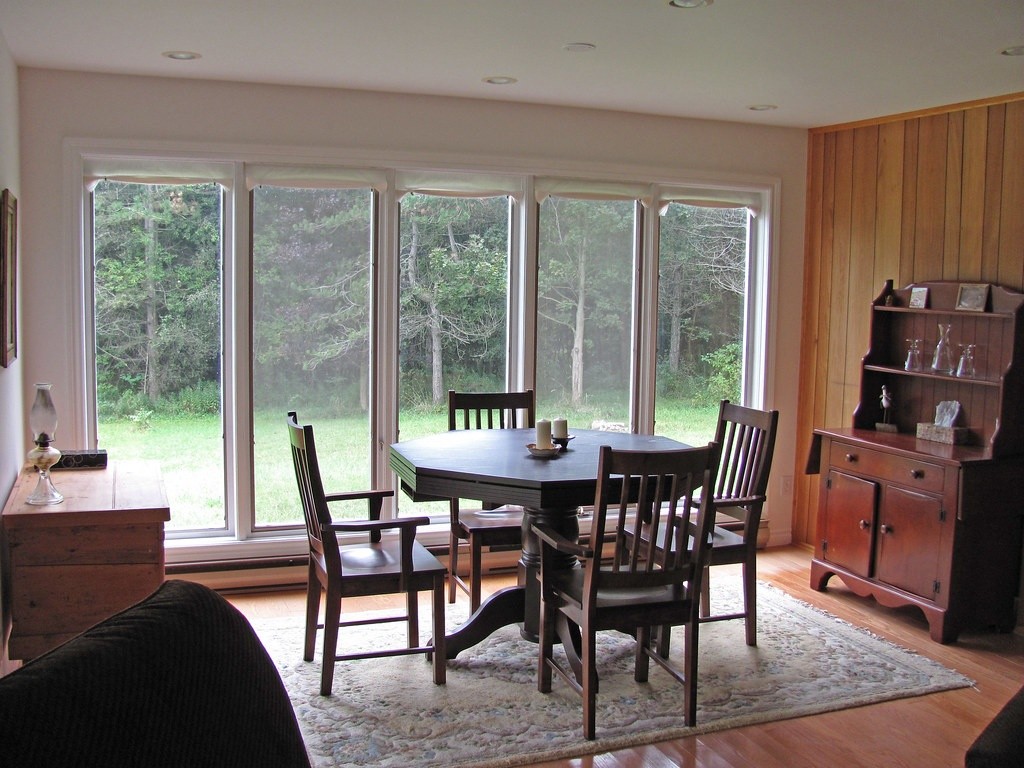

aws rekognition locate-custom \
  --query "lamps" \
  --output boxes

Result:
[25,382,64,506]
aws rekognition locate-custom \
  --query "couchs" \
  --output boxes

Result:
[0,578,313,768]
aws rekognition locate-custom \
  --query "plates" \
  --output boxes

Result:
[526,443,562,458]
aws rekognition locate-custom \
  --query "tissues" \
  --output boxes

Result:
[916,400,969,445]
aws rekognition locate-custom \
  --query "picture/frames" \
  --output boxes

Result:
[955,283,990,313]
[1,188,17,369]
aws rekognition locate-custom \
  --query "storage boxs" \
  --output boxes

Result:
[34,449,107,470]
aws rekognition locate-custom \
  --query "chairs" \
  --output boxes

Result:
[285,411,446,696]
[448,390,779,741]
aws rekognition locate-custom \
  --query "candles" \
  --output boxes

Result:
[535,418,552,450]
[552,417,567,438]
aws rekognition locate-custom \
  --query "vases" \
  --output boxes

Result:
[932,323,955,372]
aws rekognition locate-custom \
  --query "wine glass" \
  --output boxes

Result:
[904,338,923,373]
[955,343,977,380]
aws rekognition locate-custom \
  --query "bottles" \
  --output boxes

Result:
[930,323,956,375]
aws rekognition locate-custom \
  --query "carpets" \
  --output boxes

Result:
[246,579,975,768]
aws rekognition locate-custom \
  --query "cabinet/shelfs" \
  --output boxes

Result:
[805,279,1024,645]
[1,459,171,668]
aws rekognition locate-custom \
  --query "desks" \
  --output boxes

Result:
[388,428,699,693]
